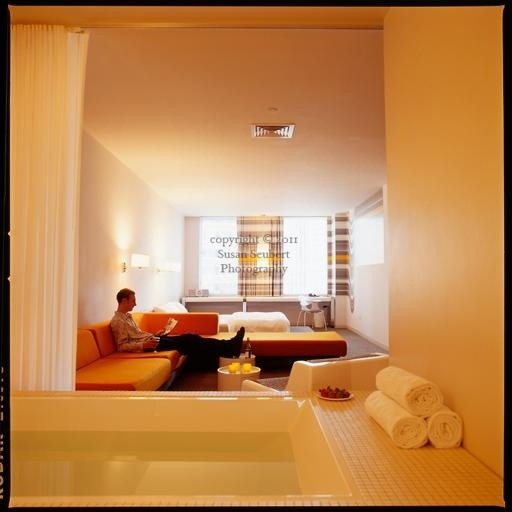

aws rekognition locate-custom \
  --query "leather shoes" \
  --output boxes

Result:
[232,327,244,359]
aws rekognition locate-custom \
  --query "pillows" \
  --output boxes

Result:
[152,301,188,313]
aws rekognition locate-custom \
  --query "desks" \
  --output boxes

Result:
[181,296,334,327]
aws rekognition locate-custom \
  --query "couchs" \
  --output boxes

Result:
[75,311,347,391]
[241,352,389,391]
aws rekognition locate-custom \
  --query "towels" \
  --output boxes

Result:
[425,403,463,448]
[364,390,428,449]
[376,366,444,418]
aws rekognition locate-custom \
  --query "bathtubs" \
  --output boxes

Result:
[11,396,362,500]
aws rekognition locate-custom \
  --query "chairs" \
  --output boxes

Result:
[296,294,327,331]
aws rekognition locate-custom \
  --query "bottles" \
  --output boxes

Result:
[245,337,252,358]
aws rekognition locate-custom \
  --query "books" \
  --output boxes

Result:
[159,316,181,337]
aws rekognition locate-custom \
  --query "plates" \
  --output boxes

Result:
[314,390,353,402]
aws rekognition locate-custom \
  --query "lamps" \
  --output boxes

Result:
[123,253,150,272]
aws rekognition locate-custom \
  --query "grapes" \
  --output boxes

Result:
[319,387,349,398]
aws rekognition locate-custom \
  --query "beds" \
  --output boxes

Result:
[143,302,290,332]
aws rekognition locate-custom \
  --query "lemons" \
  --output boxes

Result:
[228,364,251,373]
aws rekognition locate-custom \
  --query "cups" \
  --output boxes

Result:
[228,365,238,374]
[232,362,241,371]
[244,363,251,373]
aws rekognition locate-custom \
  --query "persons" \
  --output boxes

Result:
[110,287,245,357]
